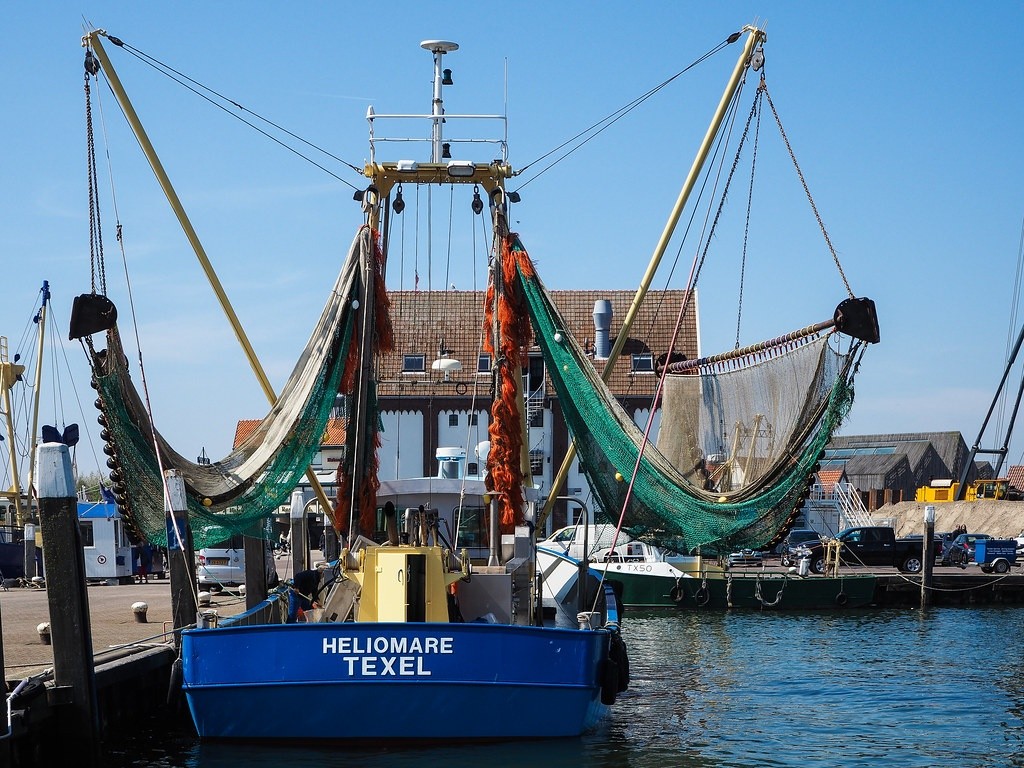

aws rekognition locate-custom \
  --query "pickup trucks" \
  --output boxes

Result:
[793,526,944,575]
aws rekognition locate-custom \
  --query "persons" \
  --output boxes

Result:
[319,530,326,559]
[286,564,328,624]
[135,541,150,584]
[951,523,969,542]
[279,528,292,554]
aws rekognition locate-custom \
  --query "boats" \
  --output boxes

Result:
[67,26,880,757]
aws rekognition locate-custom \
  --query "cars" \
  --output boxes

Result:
[781,530,822,566]
[729,549,763,569]
[946,533,994,566]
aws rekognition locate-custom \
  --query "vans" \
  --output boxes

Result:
[199,523,278,589]
[536,524,630,561]
